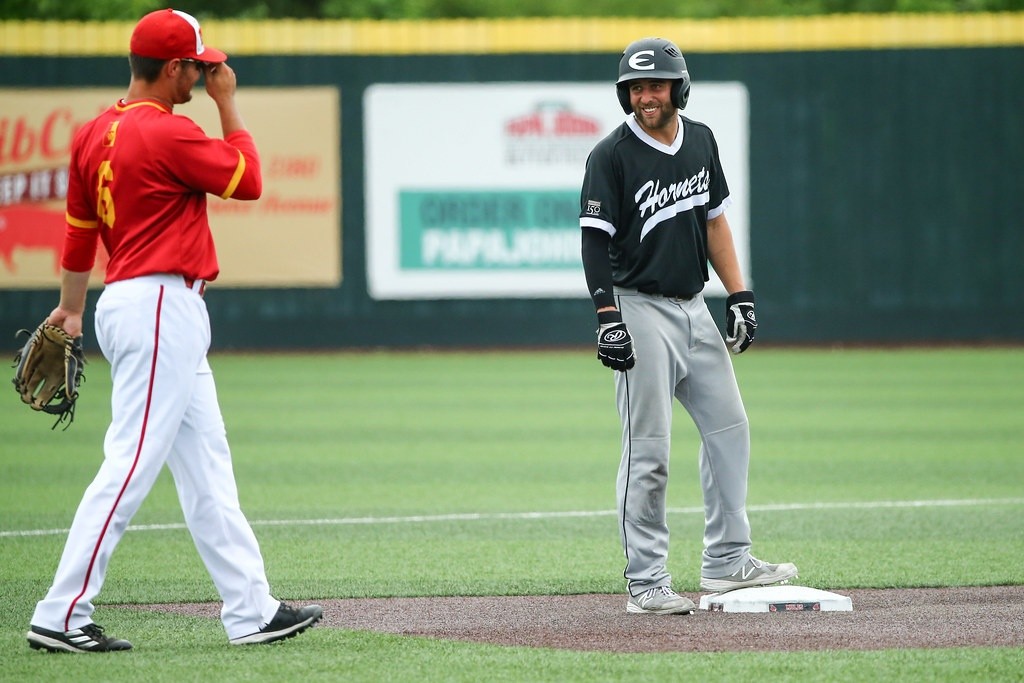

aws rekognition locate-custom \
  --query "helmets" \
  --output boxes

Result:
[615,37,691,115]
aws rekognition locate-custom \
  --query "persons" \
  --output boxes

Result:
[11,9,324,654]
[579,38,797,614]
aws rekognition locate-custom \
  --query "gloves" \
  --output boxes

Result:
[595,311,636,372]
[726,290,758,354]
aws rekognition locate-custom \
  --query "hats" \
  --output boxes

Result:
[130,8,227,62]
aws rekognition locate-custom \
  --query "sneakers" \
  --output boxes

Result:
[230,603,323,644]
[701,557,798,593]
[627,586,695,616]
[27,624,133,653]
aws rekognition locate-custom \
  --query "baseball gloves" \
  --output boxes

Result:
[12,317,86,418]
